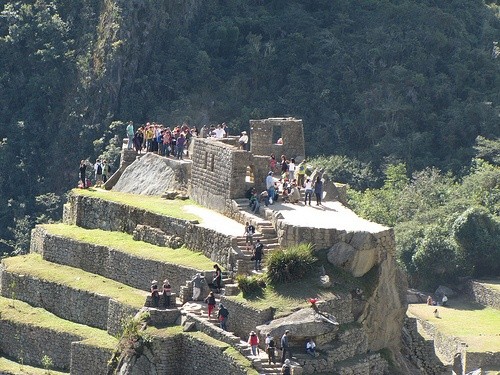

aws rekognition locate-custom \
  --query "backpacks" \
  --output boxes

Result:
[219,308,229,317]
[108,165,112,172]
[268,340,276,347]
[97,163,102,175]
[247,225,255,235]
[283,365,290,375]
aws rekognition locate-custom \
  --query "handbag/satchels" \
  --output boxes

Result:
[251,256,256,261]
[209,300,216,305]
[166,292,171,297]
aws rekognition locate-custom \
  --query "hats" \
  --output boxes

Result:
[268,170,274,175]
[152,280,157,284]
[284,359,290,364]
[163,279,169,283]
[262,191,268,196]
[241,131,247,134]
[250,331,256,335]
[210,124,215,127]
[204,125,207,128]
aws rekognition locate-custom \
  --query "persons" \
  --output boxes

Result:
[428,295,448,317]
[248,331,259,356]
[281,359,293,375]
[79,160,108,188]
[204,291,216,319]
[214,263,221,293]
[243,222,264,271]
[277,138,283,144]
[239,131,249,150]
[217,303,229,331]
[151,279,172,308]
[125,119,228,161]
[248,153,323,213]
[303,336,319,358]
[281,330,292,365]
[265,333,277,366]
